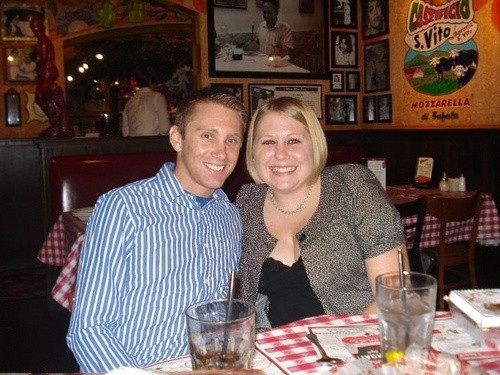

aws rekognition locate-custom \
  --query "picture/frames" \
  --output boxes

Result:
[324,0,360,125]
[248,82,323,120]
[210,83,244,104]
[207,0,329,80]
[299,0,315,13]
[213,0,247,9]
[360,0,392,123]
[256,0,280,8]
[0,6,49,41]
[2,45,46,85]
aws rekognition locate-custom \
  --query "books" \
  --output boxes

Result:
[450,288,500,328]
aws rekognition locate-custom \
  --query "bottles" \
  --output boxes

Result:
[439,178,449,191]
[459,174,465,191]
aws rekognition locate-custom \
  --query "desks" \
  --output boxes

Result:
[37,186,500,314]
[250,308,500,375]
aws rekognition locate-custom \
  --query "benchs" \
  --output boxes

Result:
[50,144,366,217]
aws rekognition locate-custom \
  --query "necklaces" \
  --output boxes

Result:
[270,184,313,215]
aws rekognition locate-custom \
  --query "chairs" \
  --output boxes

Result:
[393,197,427,282]
[433,190,482,309]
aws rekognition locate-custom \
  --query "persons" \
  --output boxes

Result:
[122,67,171,137]
[243,0,294,60]
[365,0,385,35]
[336,35,355,65]
[333,75,341,89]
[234,97,411,335]
[66,87,248,375]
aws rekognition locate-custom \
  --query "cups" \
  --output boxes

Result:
[375,271,437,375]
[185,299,256,370]
